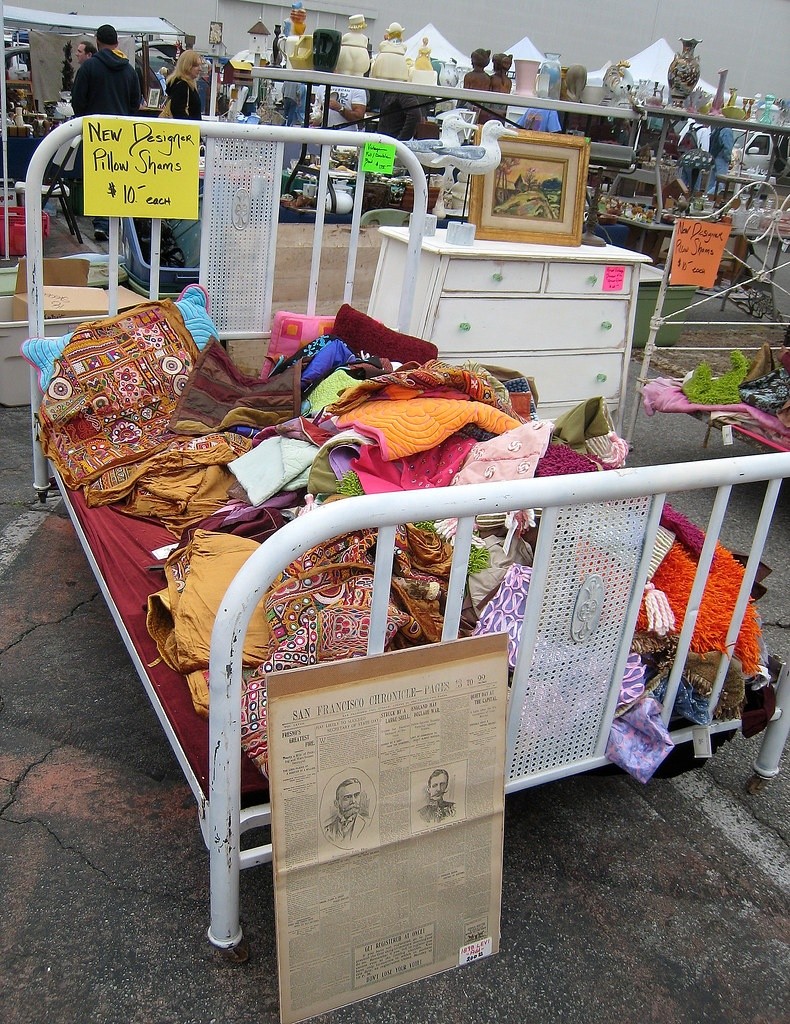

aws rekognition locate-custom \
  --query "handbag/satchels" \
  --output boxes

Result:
[157,80,189,119]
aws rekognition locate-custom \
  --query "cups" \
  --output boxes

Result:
[313,29,341,73]
[515,60,540,95]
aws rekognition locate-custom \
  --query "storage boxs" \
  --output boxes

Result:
[12,258,152,320]
[8,126,27,137]
[19,194,58,217]
[0,178,17,206]
[0,296,109,406]
[121,176,204,292]
[127,278,181,303]
[0,206,50,254]
[634,284,700,348]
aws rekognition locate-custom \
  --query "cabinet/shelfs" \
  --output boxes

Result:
[367,225,654,431]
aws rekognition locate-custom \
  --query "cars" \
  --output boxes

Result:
[730,128,774,175]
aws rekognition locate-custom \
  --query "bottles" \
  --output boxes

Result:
[733,195,750,228]
[539,53,562,100]
[762,198,775,229]
[747,200,764,229]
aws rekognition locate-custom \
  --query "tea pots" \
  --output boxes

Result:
[438,59,458,87]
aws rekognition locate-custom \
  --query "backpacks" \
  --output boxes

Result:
[678,122,705,155]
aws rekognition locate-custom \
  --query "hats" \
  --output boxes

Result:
[96,25,118,45]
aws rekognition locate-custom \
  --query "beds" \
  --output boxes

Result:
[24,113,790,951]
[623,180,790,454]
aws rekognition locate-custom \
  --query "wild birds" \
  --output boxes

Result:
[398,113,518,175]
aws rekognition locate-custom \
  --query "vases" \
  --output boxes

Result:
[734,194,751,229]
[664,37,704,111]
[538,53,563,99]
[749,198,764,229]
[759,198,774,232]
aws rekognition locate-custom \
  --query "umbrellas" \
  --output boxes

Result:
[372,24,782,117]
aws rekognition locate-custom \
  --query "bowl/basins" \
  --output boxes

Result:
[580,86,604,104]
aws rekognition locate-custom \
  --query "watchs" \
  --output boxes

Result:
[338,107,345,114]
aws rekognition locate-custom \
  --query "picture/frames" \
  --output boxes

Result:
[468,121,592,247]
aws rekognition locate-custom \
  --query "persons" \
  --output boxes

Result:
[75,40,219,110]
[489,52,512,126]
[515,106,737,193]
[71,24,140,242]
[460,48,491,124]
[279,76,421,141]
[165,50,204,122]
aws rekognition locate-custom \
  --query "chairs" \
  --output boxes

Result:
[13,134,84,245]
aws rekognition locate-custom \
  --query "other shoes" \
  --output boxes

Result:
[94,229,109,240]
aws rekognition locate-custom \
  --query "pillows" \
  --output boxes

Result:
[18,284,219,395]
[329,303,439,367]
[260,311,335,381]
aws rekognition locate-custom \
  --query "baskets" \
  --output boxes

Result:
[0,206,50,255]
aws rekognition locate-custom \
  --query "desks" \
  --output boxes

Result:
[608,105,790,227]
[248,66,647,239]
[736,171,776,186]
[599,210,790,328]
[715,174,757,218]
